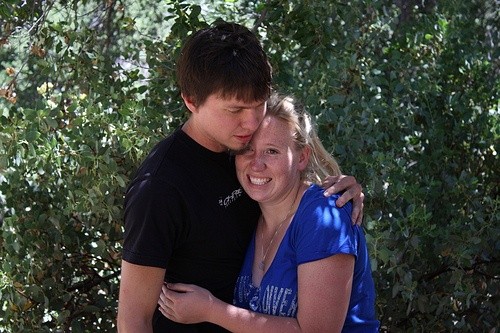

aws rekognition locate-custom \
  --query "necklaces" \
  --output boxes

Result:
[258,188,299,264]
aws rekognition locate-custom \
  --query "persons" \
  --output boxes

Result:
[117,22,364,333]
[158,93,380,333]
